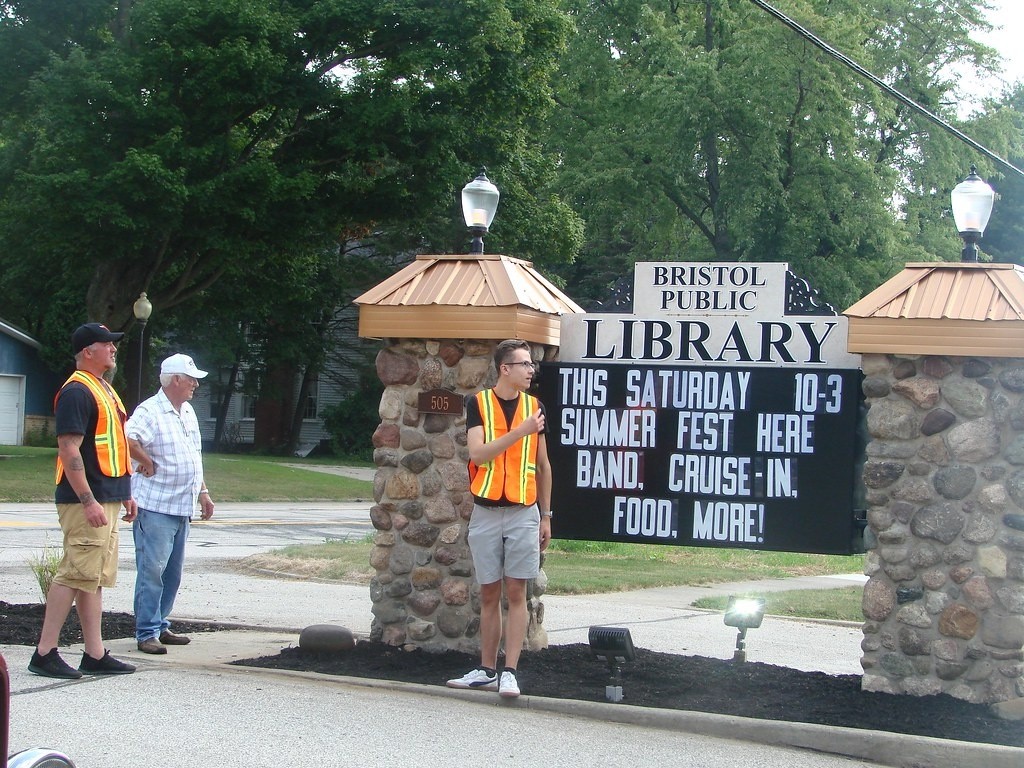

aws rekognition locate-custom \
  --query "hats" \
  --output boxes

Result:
[160,353,208,379]
[71,323,125,354]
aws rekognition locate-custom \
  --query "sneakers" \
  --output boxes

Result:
[159,629,191,645]
[78,648,136,674]
[499,671,521,696]
[138,636,168,654]
[446,669,499,692]
[28,647,82,679]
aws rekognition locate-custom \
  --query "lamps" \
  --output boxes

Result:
[950,164,997,262]
[586,625,637,702]
[461,164,501,255]
[723,596,766,664]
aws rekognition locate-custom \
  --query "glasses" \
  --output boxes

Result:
[505,361,536,371]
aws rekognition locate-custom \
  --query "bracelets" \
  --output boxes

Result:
[541,511,552,518]
[201,488,209,494]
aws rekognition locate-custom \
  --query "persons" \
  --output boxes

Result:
[27,323,138,679]
[124,354,216,654]
[445,338,552,693]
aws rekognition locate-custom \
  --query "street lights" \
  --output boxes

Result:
[134,292,152,408]
